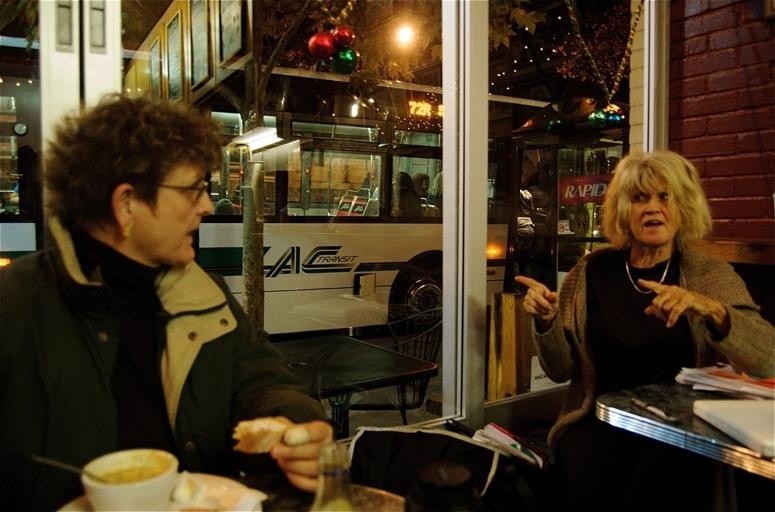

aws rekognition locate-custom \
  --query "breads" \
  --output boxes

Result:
[233,416,291,454]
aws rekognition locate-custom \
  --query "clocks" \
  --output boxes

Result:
[12,121,29,137]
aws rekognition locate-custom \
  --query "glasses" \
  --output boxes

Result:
[156,180,209,201]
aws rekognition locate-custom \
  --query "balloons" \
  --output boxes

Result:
[589,109,629,131]
[333,47,358,76]
[330,26,355,52]
[309,31,336,61]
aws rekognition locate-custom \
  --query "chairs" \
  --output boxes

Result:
[332,186,379,217]
[348,307,443,426]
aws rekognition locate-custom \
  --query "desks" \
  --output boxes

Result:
[596,366,775,481]
[270,334,438,439]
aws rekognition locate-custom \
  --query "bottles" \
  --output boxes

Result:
[307,444,358,511]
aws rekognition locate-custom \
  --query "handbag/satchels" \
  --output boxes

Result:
[347,417,545,511]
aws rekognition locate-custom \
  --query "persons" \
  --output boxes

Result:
[517,177,535,294]
[10,182,20,204]
[392,173,422,217]
[0,93,335,511]
[428,173,443,205]
[514,149,775,512]
[412,172,430,204]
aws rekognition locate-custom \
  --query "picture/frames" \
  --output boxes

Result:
[146,35,162,104]
[163,9,185,107]
[186,0,214,93]
[213,0,250,70]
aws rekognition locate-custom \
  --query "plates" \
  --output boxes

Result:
[53,471,264,512]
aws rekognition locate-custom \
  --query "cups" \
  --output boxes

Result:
[81,447,179,512]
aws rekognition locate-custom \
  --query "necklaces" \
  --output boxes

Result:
[626,257,671,294]
